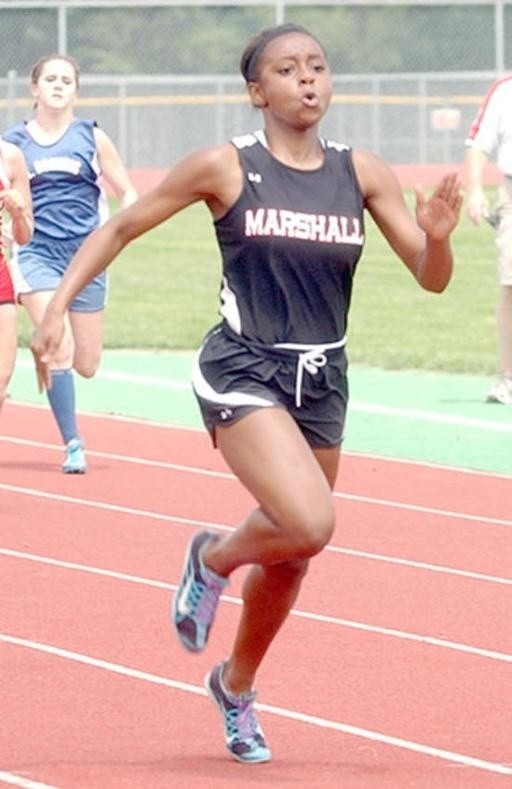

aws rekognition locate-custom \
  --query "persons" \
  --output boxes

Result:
[3,51,141,476]
[1,132,37,432]
[26,18,467,767]
[463,70,512,405]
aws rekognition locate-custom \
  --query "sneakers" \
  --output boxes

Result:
[482,374,512,405]
[61,439,86,474]
[172,527,230,653]
[204,658,272,764]
